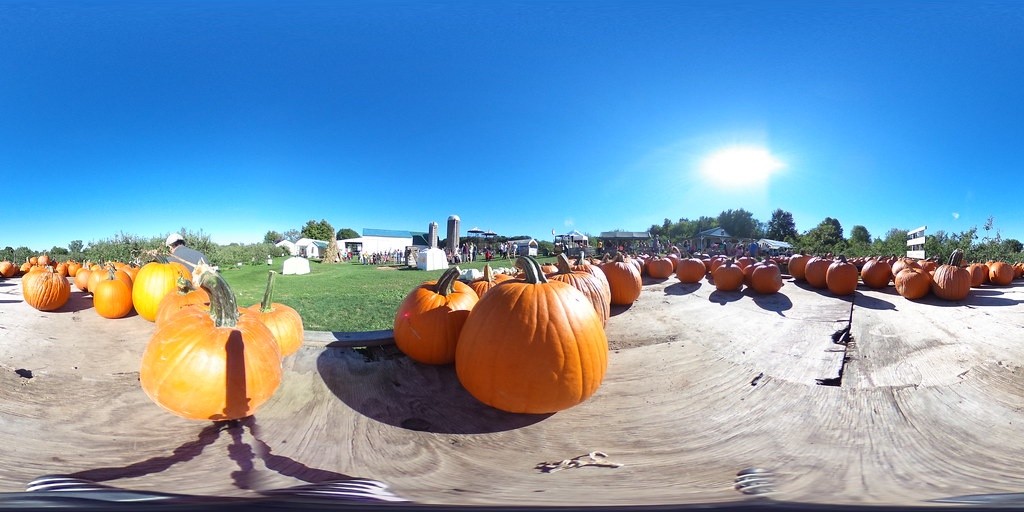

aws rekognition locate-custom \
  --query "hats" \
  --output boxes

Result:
[166,233,184,248]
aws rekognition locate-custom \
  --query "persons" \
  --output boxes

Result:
[597,235,834,260]
[166,233,212,288]
[450,241,519,264]
[342,249,404,264]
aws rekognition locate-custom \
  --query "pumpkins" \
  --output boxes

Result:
[0,252,304,421]
[394,249,1024,414]
